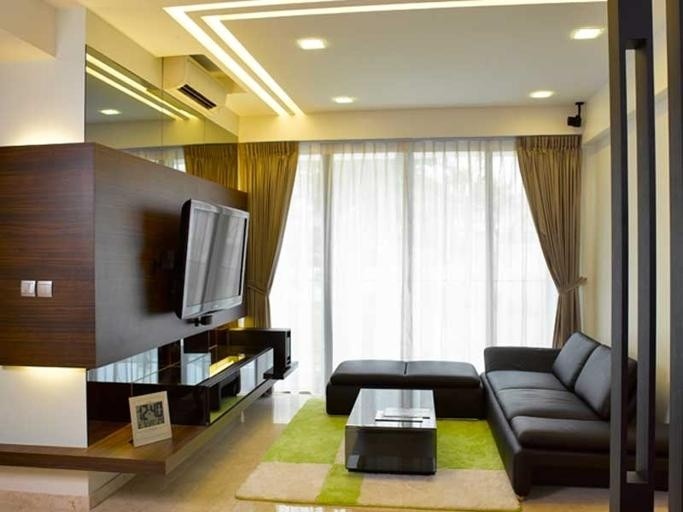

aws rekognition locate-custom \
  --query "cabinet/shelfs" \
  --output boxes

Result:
[88,325,300,475]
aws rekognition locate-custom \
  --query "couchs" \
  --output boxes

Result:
[479,332,669,501]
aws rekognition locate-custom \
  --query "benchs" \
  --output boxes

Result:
[326,360,485,419]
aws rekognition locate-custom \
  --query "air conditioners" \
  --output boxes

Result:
[165,56,228,120]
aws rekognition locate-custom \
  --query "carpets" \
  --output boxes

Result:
[235,398,519,511]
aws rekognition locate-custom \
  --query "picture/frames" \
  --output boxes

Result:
[129,391,172,448]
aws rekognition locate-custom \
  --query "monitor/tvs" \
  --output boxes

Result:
[171,198,250,321]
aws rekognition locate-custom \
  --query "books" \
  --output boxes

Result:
[375,406,430,422]
